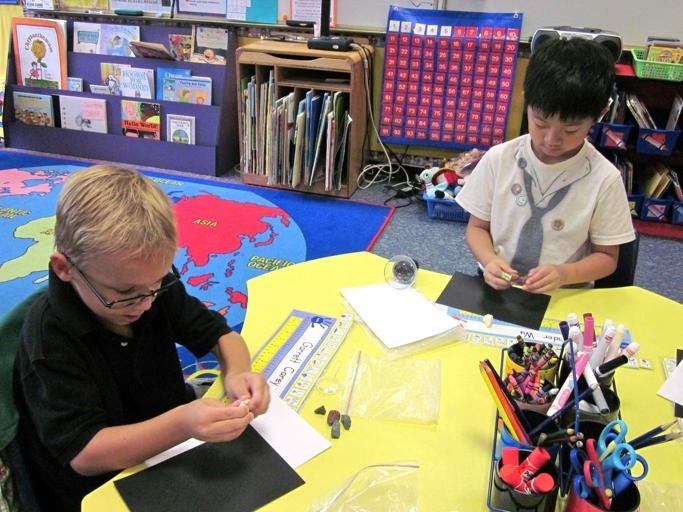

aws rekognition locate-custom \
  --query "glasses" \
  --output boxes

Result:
[61,251,181,311]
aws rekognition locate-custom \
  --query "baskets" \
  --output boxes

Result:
[631,49,683,82]
[422,189,470,223]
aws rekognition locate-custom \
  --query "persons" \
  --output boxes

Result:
[454,37,637,294]
[12,164,270,512]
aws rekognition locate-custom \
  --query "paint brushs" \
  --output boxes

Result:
[549,311,638,418]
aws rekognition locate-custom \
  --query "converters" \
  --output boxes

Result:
[397,187,414,198]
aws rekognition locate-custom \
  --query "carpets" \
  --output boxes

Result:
[0,141,398,380]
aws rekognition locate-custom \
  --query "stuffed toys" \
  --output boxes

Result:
[415,162,465,198]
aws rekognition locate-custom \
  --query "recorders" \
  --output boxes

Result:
[530,25,623,64]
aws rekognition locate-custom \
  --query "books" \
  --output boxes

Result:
[607,150,683,201]
[643,35,683,80]
[598,82,683,131]
[240,69,353,192]
[12,16,230,146]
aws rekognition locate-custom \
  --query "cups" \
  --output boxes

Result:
[492,341,640,511]
[383,255,417,292]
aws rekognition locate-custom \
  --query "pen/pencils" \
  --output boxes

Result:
[479,358,600,449]
[505,282,529,291]
[499,447,554,496]
[630,413,681,449]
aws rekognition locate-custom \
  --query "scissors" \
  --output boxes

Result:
[570,419,646,503]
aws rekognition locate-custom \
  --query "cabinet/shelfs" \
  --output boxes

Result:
[3,19,240,177]
[233,39,376,202]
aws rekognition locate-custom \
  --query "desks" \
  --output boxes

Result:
[73,250,683,512]
